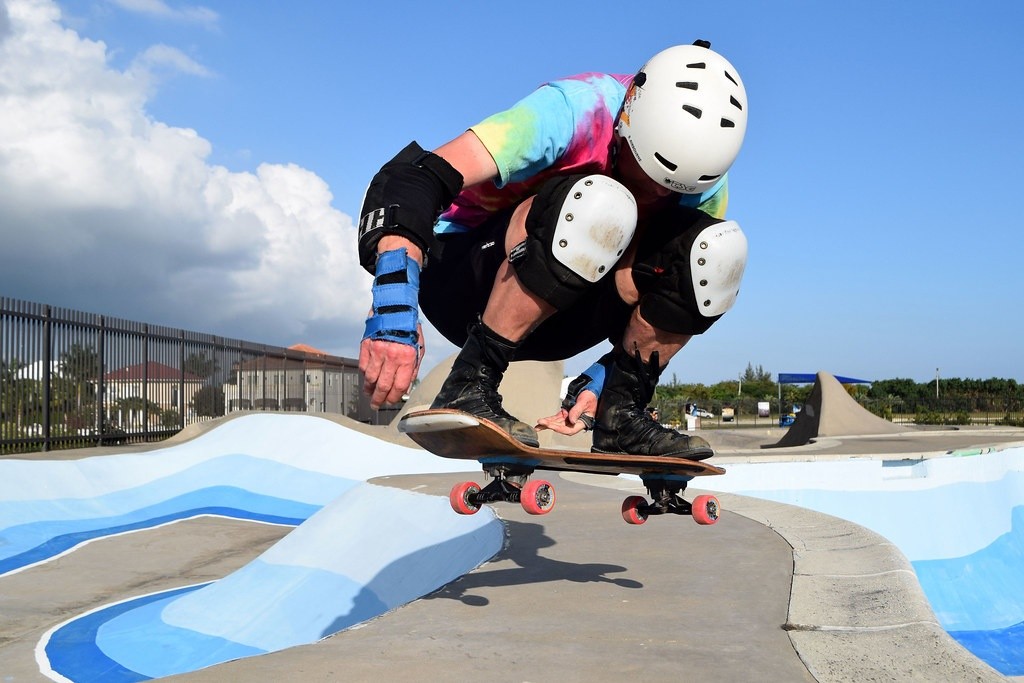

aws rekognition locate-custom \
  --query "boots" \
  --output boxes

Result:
[430,318,541,450]
[590,341,714,459]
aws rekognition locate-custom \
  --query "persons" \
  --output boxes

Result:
[358,43,748,461]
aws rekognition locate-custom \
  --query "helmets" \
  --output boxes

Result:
[614,44,747,194]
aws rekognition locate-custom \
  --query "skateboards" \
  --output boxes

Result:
[398,409,729,525]
